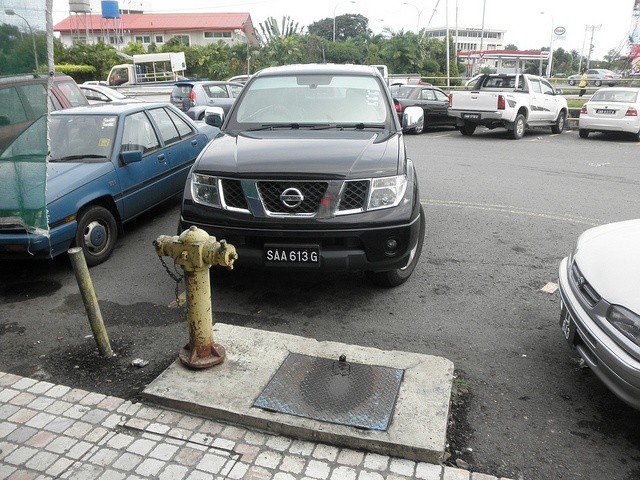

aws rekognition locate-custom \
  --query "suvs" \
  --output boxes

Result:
[177,63,427,290]
[170,79,244,121]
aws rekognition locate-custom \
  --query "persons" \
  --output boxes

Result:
[579,66,589,97]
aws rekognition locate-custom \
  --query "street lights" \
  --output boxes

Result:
[538,9,556,78]
[5,9,39,71]
[331,0,357,43]
[402,2,432,37]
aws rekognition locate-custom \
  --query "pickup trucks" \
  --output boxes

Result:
[369,63,422,85]
[447,74,566,137]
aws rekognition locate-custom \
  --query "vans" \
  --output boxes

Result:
[1,74,91,153]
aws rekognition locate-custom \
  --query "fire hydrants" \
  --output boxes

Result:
[151,226,239,370]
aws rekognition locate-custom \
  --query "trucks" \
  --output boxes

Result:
[106,52,187,87]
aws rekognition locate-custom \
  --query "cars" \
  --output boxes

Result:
[558,218,640,408]
[76,83,144,104]
[391,83,457,130]
[579,87,638,142]
[567,68,622,87]
[1,101,223,267]
